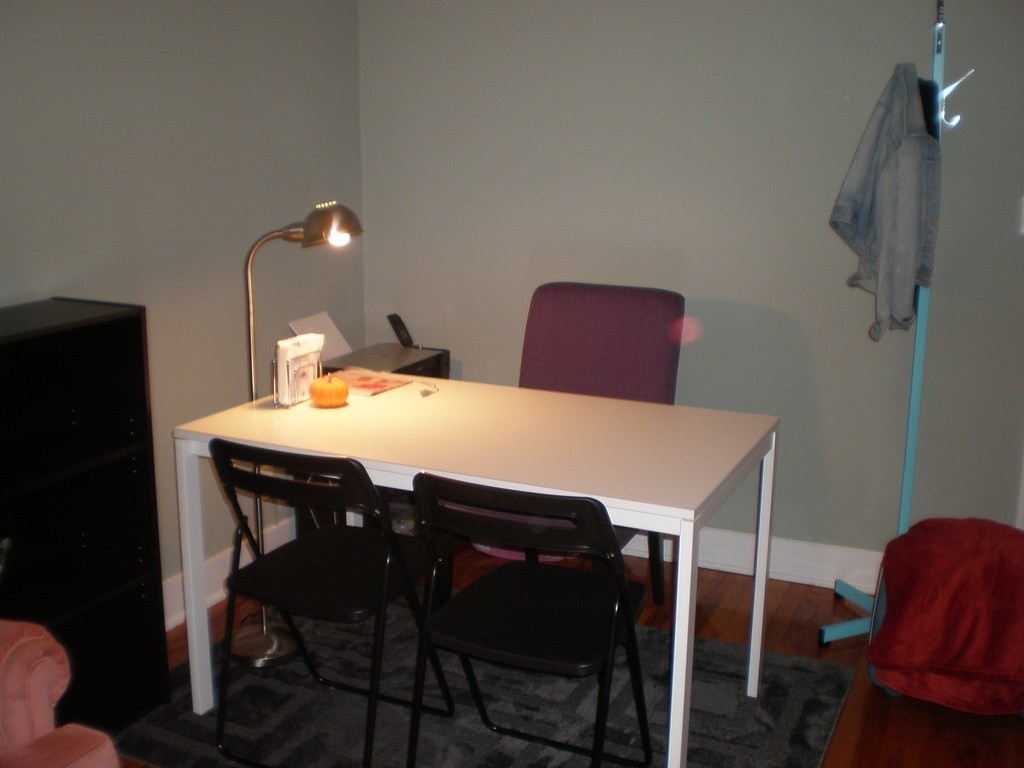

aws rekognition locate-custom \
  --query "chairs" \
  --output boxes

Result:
[469,278,687,605]
[208,439,455,768]
[404,469,656,768]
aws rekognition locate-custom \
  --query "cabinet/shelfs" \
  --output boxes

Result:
[320,341,451,378]
[0,293,176,735]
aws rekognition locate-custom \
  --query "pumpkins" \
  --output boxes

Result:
[309,372,348,407]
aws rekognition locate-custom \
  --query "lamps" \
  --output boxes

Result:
[228,201,365,668]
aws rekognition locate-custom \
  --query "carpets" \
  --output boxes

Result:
[112,607,861,768]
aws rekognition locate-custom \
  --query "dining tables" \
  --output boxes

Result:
[171,362,779,768]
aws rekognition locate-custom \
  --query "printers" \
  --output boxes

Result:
[288,312,450,379]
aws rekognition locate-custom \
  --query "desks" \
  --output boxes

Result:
[817,652,1024,768]
